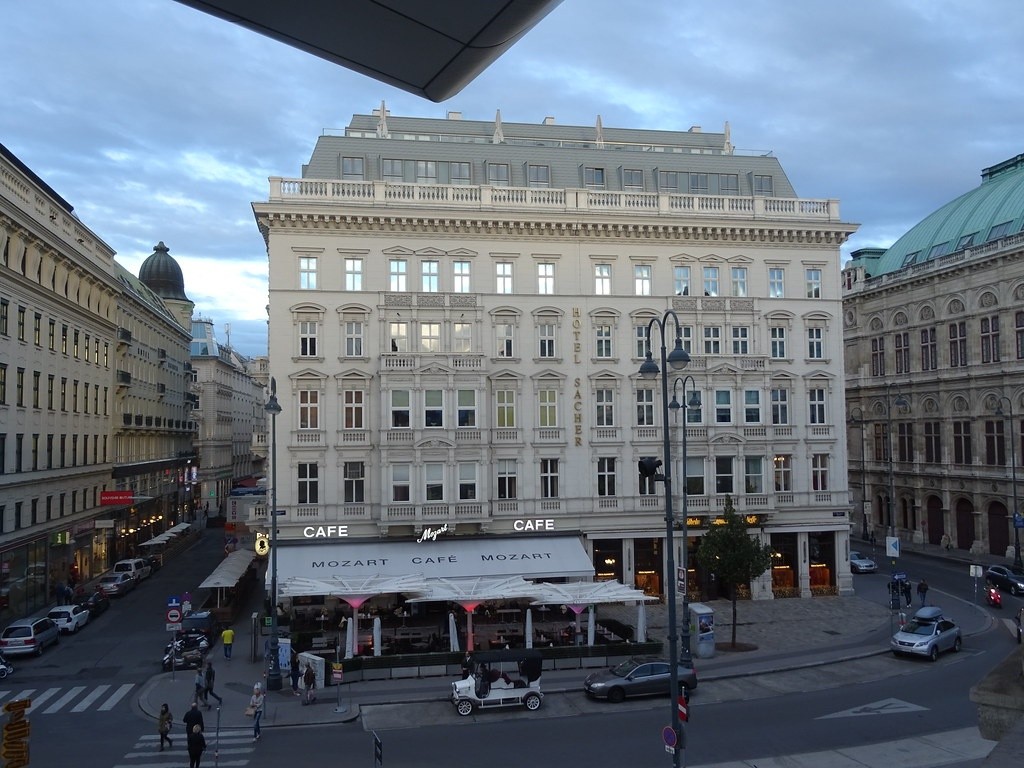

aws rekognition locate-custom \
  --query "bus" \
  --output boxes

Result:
[24,562,46,584]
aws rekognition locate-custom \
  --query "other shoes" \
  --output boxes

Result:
[208,705,211,711]
[907,605,910,608]
[224,655,228,658]
[159,747,164,752]
[219,697,222,704]
[909,604,912,607]
[169,739,173,747]
[228,657,231,659]
[256,735,260,739]
[297,689,301,692]
[201,705,207,707]
[253,738,256,742]
[294,691,301,696]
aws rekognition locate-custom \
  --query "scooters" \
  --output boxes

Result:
[983,586,1003,609]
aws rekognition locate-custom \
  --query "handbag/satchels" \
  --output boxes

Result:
[245,707,255,716]
[166,713,171,731]
[308,689,316,700]
[205,680,213,688]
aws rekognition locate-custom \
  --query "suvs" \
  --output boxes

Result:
[889,606,962,662]
[0,617,61,657]
[49,573,135,634]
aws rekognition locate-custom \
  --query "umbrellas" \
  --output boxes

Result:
[138,522,191,546]
[198,548,257,609]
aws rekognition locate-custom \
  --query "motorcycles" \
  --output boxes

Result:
[162,629,210,671]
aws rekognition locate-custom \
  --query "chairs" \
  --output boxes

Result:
[533,626,592,645]
[380,631,436,653]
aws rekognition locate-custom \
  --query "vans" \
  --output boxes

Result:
[114,559,152,582]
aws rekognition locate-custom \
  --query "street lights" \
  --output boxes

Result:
[638,307,705,768]
[886,380,908,537]
[851,407,872,541]
[265,378,283,692]
[994,396,1022,565]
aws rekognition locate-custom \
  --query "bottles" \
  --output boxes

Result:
[404,611,407,616]
[550,643,553,647]
[541,634,543,641]
[500,636,503,642]
[604,625,610,634]
[506,644,509,650]
[368,612,371,618]
[597,623,600,629]
[626,639,630,643]
[322,615,324,620]
[611,632,614,638]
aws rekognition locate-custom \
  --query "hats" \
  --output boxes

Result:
[254,682,261,689]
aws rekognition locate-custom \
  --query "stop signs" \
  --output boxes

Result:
[679,696,687,721]
[166,608,183,624]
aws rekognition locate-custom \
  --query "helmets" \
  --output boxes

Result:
[986,580,991,586]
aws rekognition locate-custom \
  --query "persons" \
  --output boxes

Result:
[301,662,313,705]
[187,724,207,768]
[0,649,14,669]
[157,703,173,752]
[250,688,264,742]
[290,653,301,696]
[194,668,212,711]
[55,582,74,606]
[221,626,236,660]
[943,533,952,551]
[203,662,222,704]
[916,578,929,608]
[461,651,490,697]
[183,703,205,752]
[903,578,912,608]
[201,501,210,520]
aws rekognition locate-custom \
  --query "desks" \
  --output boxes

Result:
[393,634,422,648]
[490,639,512,650]
[551,622,573,643]
[579,621,623,643]
[495,630,524,638]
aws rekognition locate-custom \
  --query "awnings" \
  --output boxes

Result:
[265,533,595,590]
[238,478,257,487]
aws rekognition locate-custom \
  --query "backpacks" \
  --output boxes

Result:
[64,588,70,597]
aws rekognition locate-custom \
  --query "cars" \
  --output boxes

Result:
[584,657,697,703]
[450,650,545,717]
[0,648,14,679]
[0,577,25,608]
[850,551,880,572]
[1014,605,1024,643]
[983,562,1024,595]
